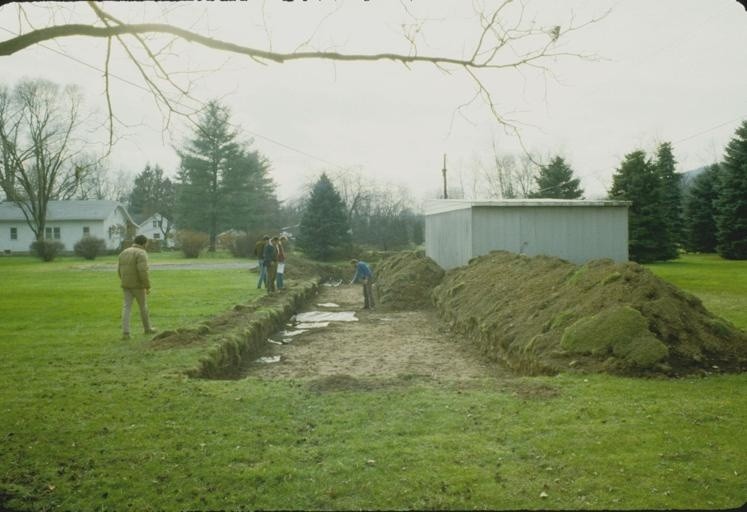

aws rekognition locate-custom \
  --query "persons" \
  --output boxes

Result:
[254,235,289,296]
[116,234,157,342]
[349,259,377,312]
